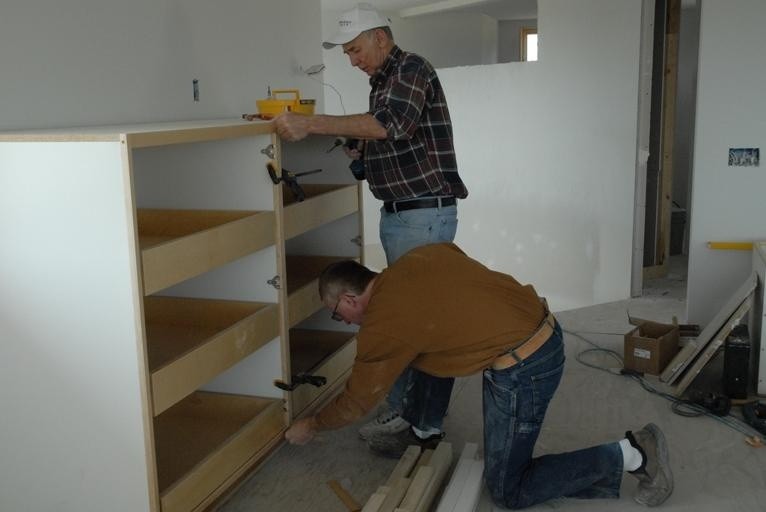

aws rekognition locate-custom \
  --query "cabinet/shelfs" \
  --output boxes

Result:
[0,118,368,512]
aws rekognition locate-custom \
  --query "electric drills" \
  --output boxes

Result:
[325,136,353,154]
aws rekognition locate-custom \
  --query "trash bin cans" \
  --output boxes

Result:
[724,323,750,399]
[672,206,687,257]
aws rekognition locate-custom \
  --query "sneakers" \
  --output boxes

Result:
[358,409,446,458]
[624,422,674,508]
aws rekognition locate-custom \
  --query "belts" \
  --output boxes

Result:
[493,311,555,371]
[383,197,456,214]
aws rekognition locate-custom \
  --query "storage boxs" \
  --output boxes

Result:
[624,320,678,374]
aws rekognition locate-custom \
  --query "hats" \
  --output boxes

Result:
[320,6,393,50]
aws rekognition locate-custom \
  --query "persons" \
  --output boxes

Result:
[283,242,673,509]
[273,10,469,459]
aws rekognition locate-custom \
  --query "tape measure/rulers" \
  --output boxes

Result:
[325,477,365,512]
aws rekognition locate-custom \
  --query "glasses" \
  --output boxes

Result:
[330,295,356,322]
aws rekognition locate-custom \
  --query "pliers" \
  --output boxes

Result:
[242,114,272,121]
